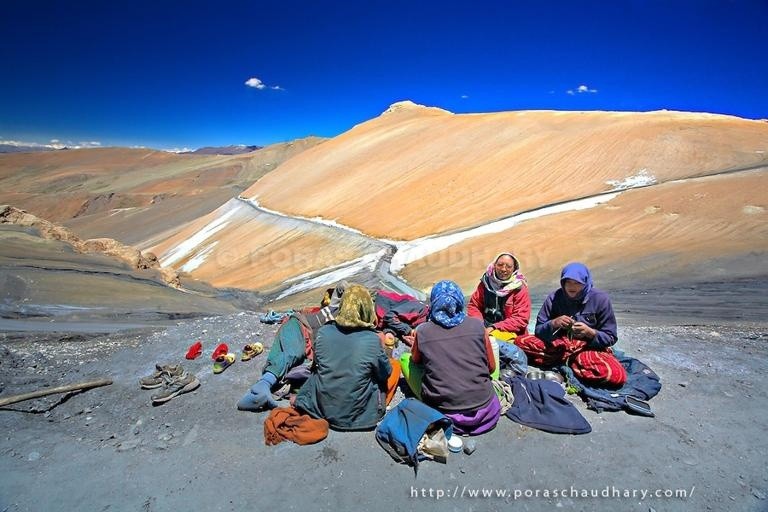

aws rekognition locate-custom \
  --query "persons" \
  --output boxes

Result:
[236,251,618,437]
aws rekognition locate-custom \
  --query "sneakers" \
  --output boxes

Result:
[240,341,265,362]
[185,341,204,360]
[259,310,283,324]
[212,343,230,362]
[150,369,201,404]
[137,360,186,390]
[211,352,237,374]
